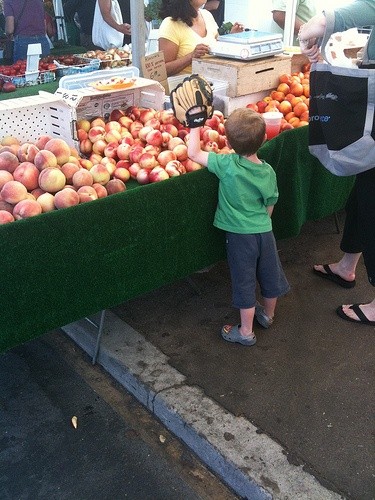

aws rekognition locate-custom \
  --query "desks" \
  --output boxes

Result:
[0,124,358,366]
[0,76,63,100]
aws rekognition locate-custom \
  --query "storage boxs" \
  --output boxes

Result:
[0,89,75,150]
[214,88,275,118]
[190,53,292,99]
[165,72,230,95]
[55,55,102,76]
[0,68,57,88]
[74,54,132,70]
[55,76,166,159]
[147,29,159,55]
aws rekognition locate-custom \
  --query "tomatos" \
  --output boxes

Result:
[0,56,86,92]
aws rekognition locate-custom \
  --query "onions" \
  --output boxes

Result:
[82,48,130,68]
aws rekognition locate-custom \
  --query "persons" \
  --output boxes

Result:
[3,0,50,64]
[0,0,225,78]
[186,105,292,346]
[297,0,375,324]
[270,0,317,46]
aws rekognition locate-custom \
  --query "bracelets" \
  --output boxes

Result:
[296,24,316,46]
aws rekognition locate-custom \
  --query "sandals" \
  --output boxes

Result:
[221,323,257,345]
[253,300,272,329]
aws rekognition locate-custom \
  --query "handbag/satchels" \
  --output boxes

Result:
[309,9,374,176]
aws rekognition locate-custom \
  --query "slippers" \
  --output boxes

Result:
[313,264,356,288]
[337,303,375,326]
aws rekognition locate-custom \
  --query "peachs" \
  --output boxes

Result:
[76,106,229,183]
[245,63,312,133]
[0,136,125,225]
